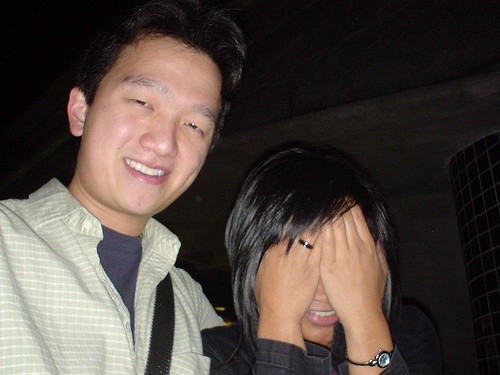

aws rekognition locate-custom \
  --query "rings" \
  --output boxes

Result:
[299,238,313,249]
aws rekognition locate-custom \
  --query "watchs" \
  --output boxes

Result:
[345,345,396,367]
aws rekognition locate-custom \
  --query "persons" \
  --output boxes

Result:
[0,0,245,375]
[201,141,441,375]
